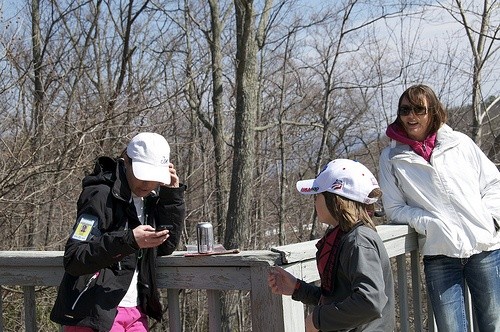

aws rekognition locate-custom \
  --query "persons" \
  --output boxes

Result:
[379,85,500,332]
[268,159,395,332]
[49,132,187,332]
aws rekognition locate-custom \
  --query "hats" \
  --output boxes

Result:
[297,159,380,204]
[127,132,171,186]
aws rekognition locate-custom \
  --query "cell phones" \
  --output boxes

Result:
[155,225,173,233]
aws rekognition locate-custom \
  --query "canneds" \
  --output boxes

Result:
[196,221,213,254]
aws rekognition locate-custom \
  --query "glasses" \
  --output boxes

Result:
[397,104,429,116]
